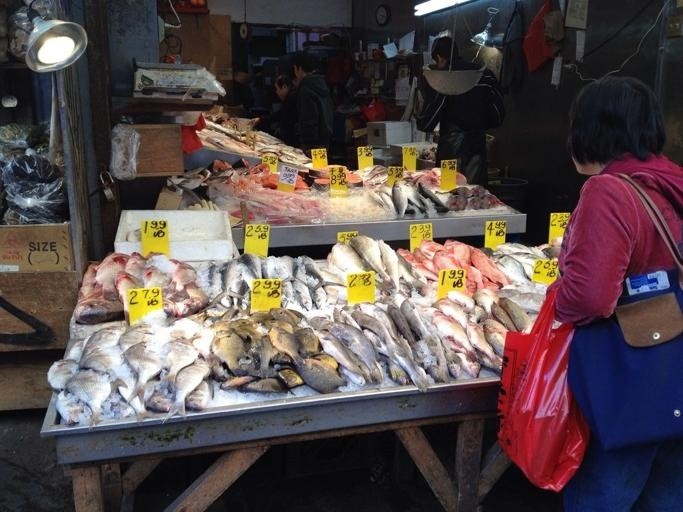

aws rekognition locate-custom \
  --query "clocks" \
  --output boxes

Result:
[375,4,390,27]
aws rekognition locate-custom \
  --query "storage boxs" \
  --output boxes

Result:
[0,200,89,418]
[114,120,184,178]
[113,208,231,261]
[231,238,241,262]
[367,119,412,149]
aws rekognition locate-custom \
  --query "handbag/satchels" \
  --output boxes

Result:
[568,266,683,451]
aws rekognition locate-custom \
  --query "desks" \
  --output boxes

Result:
[55,409,536,512]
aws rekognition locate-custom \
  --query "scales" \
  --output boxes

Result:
[133,56,227,101]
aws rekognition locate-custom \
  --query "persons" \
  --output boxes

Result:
[274,75,295,103]
[291,56,334,155]
[415,35,505,191]
[542,73,681,511]
[229,66,255,109]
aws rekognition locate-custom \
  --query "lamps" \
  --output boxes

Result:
[412,1,481,19]
[20,0,87,75]
[469,7,500,49]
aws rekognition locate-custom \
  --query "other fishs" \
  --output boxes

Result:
[376,179,450,219]
[47,234,563,430]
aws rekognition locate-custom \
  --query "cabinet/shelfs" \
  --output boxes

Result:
[157,9,234,82]
